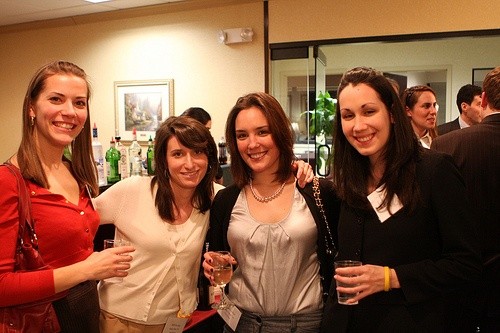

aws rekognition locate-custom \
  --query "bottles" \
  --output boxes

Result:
[91,120,158,188]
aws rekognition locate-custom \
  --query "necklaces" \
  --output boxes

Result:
[0,59,137,333]
[245,175,286,203]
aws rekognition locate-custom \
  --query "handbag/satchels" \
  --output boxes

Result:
[1,162,58,333]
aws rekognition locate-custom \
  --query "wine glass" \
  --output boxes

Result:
[208,251,235,311]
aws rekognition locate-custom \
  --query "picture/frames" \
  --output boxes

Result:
[114,78,176,146]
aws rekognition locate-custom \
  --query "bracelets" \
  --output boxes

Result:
[382,263,392,294]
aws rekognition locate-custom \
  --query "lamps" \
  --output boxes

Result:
[217,25,254,42]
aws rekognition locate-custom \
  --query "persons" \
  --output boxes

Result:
[431,63,499,333]
[436,84,485,131]
[178,106,225,186]
[326,62,478,333]
[198,91,341,333]
[399,85,444,152]
[93,113,316,333]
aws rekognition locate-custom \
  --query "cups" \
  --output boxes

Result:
[334,260,361,305]
[102,238,130,284]
